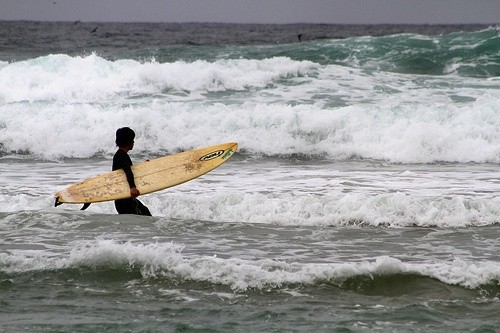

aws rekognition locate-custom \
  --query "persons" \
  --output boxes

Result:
[111,127,152,217]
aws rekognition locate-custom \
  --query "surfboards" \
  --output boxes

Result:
[54,142,238,210]
